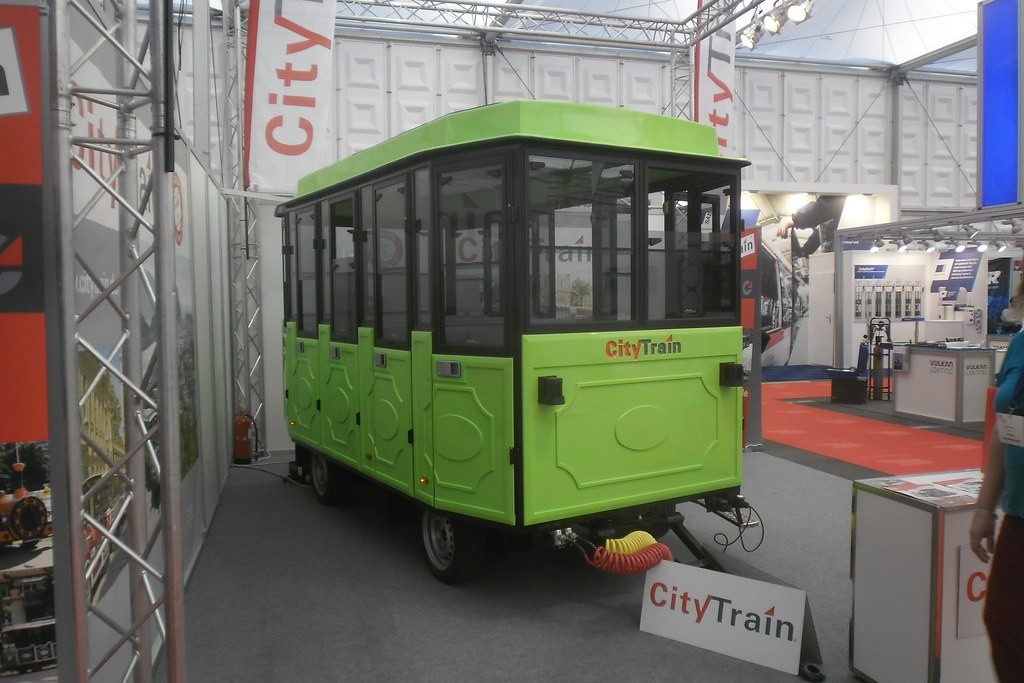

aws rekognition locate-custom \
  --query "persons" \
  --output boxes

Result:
[776,195,874,258]
[970,266,1024,683]
[1001,296,1022,335]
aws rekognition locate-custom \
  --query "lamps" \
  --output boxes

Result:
[996,240,1007,254]
[954,242,965,252]
[902,234,914,244]
[1010,220,1024,235]
[868,240,880,255]
[976,242,988,253]
[874,234,885,248]
[897,242,908,252]
[925,242,936,253]
[740,0,815,52]
[965,225,979,239]
[932,229,945,242]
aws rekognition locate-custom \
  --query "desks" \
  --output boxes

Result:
[847,467,1003,682]
[891,341,998,427]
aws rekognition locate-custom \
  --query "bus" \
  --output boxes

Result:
[281,99,754,586]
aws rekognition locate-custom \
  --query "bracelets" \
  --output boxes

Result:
[974,509,998,520]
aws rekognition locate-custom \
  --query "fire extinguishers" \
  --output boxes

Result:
[234,407,258,465]
